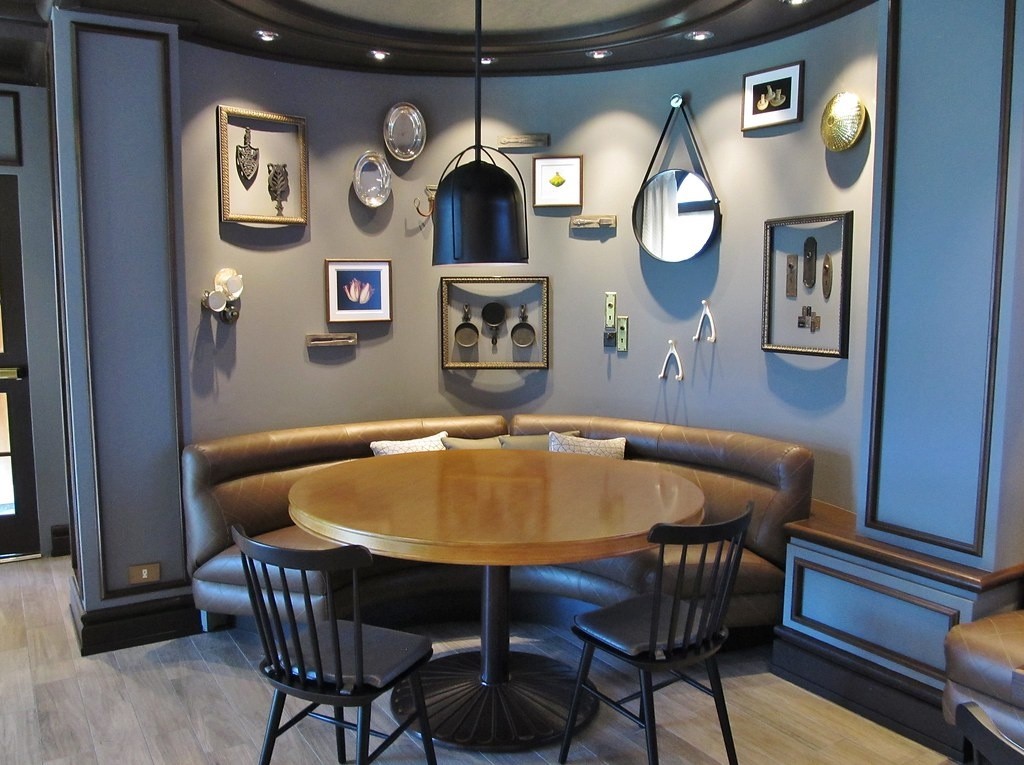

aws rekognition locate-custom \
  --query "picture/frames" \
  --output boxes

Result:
[741,59,806,132]
[324,258,393,324]
[761,210,855,358]
[440,276,549,370]
[532,154,584,210]
[217,104,309,227]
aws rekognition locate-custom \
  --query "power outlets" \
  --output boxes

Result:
[129,562,160,585]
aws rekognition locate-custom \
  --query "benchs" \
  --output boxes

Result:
[512,412,815,653]
[182,414,507,634]
[942,608,1024,750]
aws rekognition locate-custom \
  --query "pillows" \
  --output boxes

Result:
[441,434,510,451]
[499,429,581,450]
[549,430,627,461]
[369,430,448,456]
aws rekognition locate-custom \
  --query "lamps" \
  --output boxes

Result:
[430,0,533,266]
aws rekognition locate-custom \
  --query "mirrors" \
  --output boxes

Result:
[632,93,723,263]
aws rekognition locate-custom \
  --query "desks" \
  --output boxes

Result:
[286,452,705,755]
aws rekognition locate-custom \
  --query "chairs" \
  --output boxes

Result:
[953,701,1024,765]
[229,523,439,765]
[559,499,754,765]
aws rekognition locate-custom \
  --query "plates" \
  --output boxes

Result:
[352,150,392,209]
[382,101,428,161]
